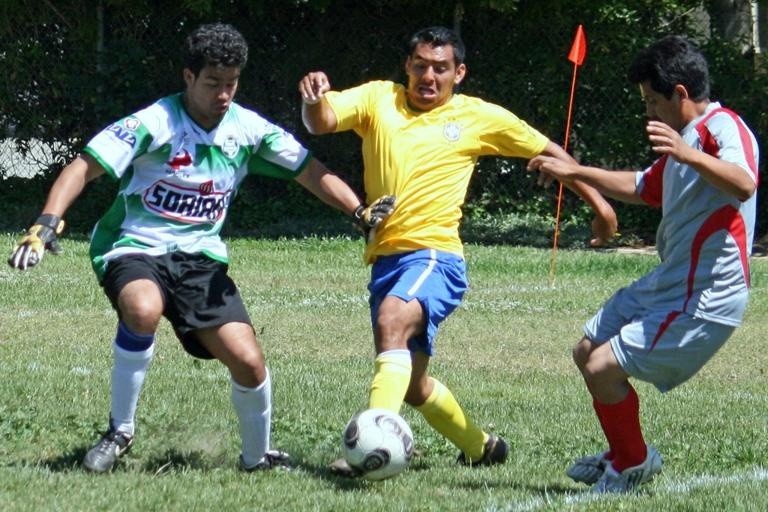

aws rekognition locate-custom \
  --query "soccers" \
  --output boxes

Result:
[341,409,414,479]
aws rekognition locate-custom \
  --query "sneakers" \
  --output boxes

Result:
[566,446,664,492]
[330,457,357,478]
[239,450,292,472]
[453,436,508,467]
[82,429,134,473]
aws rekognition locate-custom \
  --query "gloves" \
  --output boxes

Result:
[352,195,396,232]
[8,213,65,270]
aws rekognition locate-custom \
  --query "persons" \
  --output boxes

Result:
[525,34,761,496]
[297,27,618,477]
[8,23,398,474]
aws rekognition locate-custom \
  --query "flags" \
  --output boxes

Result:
[567,25,588,67]
